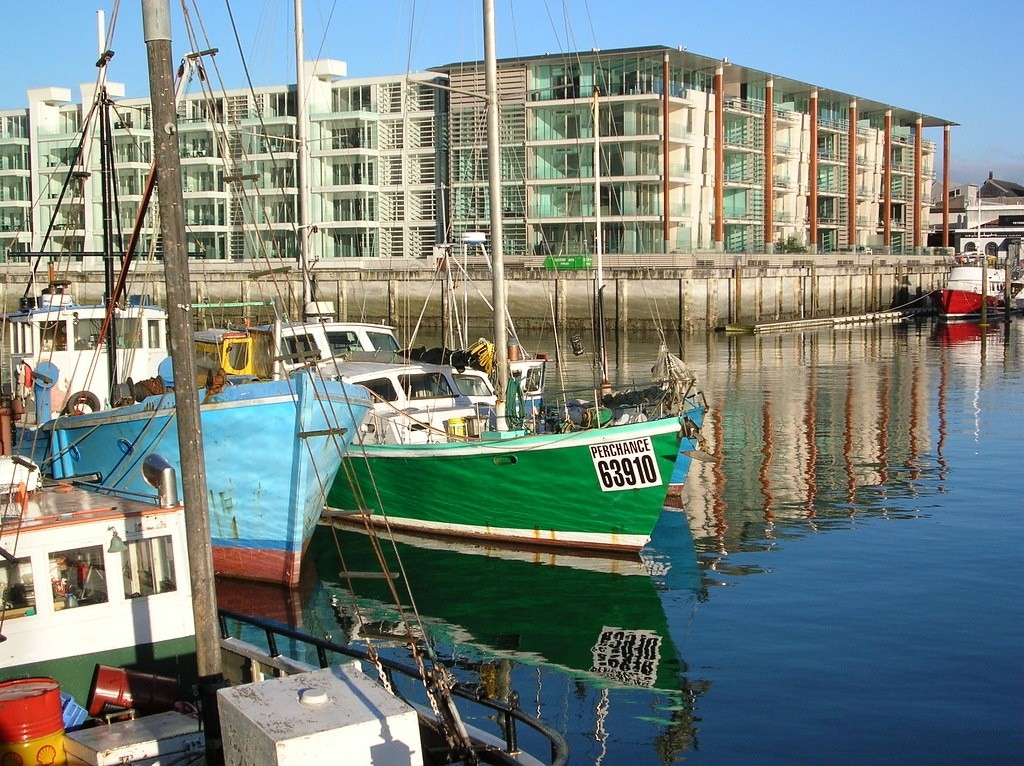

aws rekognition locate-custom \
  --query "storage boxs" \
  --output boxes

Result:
[59,690,89,735]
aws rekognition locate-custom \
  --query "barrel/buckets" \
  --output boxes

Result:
[0,679,66,766]
[448,419,464,443]
[88,664,179,716]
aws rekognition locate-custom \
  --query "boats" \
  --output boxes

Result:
[942,187,1024,320]
[2,77,710,766]
[311,515,710,765]
[1015,274,1024,311]
[640,496,709,654]
[211,553,334,669]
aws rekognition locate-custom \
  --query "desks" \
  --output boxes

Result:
[0,600,65,621]
